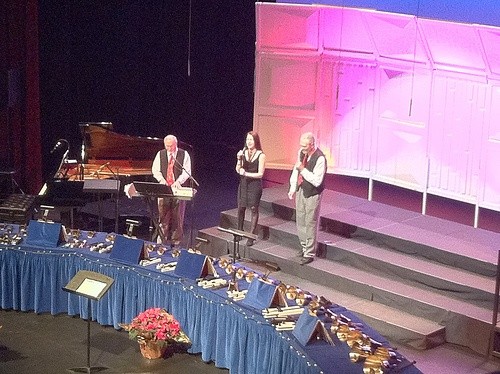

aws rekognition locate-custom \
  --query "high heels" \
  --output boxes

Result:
[233,230,244,241]
[245,234,256,246]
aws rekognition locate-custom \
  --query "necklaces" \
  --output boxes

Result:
[248,148,258,163]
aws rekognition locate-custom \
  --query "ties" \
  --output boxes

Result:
[167,154,173,185]
[297,153,308,186]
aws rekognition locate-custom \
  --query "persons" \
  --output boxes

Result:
[288,132,326,266]
[232,131,266,246]
[151,135,191,252]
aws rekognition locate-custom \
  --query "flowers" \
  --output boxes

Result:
[118,307,192,348]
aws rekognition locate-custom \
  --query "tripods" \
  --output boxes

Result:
[61,270,114,374]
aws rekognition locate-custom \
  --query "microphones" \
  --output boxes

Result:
[242,146,247,153]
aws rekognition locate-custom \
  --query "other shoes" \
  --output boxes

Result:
[300,257,313,265]
[296,249,304,257]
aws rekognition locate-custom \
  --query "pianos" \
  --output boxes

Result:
[124,181,197,247]
[47,122,187,242]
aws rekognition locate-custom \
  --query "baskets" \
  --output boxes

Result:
[118,324,190,359]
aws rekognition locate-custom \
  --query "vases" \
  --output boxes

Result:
[138,338,168,359]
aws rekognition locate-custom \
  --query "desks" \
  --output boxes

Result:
[0,223,423,374]
